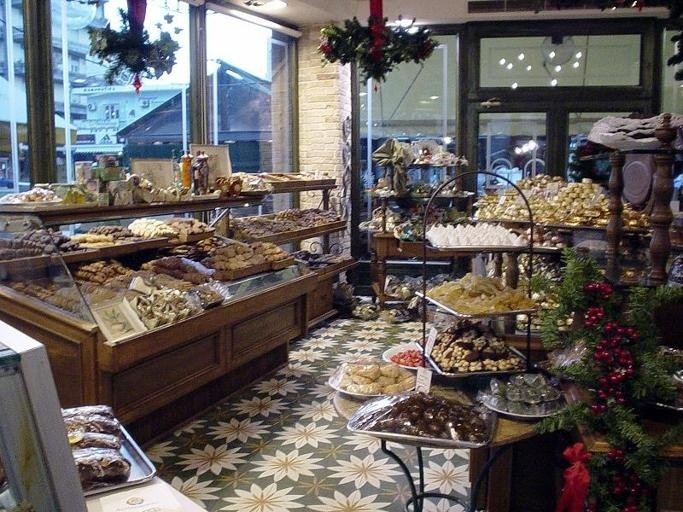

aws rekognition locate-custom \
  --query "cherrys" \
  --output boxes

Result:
[584,282,651,512]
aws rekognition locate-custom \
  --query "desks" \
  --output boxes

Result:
[554,373,683,511]
[331,363,569,510]
[78,464,210,511]
[464,309,592,511]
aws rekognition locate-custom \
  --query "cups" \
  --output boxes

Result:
[95,192,109,207]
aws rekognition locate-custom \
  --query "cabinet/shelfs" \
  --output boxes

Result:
[470,209,653,301]
[410,168,541,388]
[366,155,477,312]
[0,188,320,455]
[220,171,363,345]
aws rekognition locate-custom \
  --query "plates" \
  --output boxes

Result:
[484,400,567,421]
[384,343,433,371]
[328,366,418,402]
[0,198,62,208]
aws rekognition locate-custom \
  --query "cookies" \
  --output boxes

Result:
[141,255,216,283]
[71,233,115,248]
[152,237,228,261]
[2,227,79,260]
[64,173,179,207]
[89,225,143,241]
[125,270,225,328]
[229,214,290,238]
[6,258,136,313]
[3,187,57,202]
[338,332,561,442]
[165,217,215,242]
[205,240,291,279]
[128,218,178,239]
[231,172,272,193]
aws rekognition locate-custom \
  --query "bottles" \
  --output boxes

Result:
[74,161,92,182]
[170,149,210,194]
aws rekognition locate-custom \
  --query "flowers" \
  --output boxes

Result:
[313,12,443,88]
